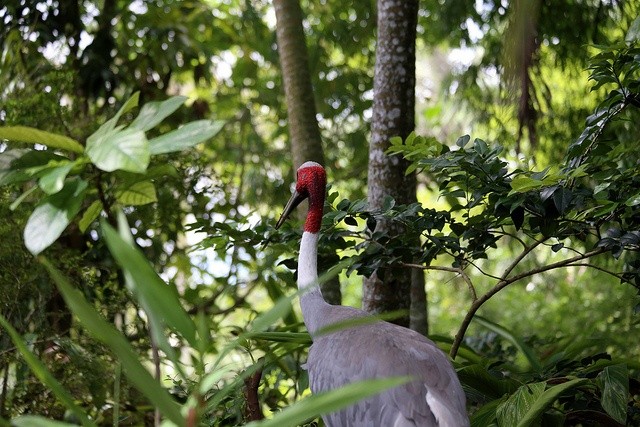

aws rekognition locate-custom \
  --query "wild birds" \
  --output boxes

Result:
[273,159,473,427]
